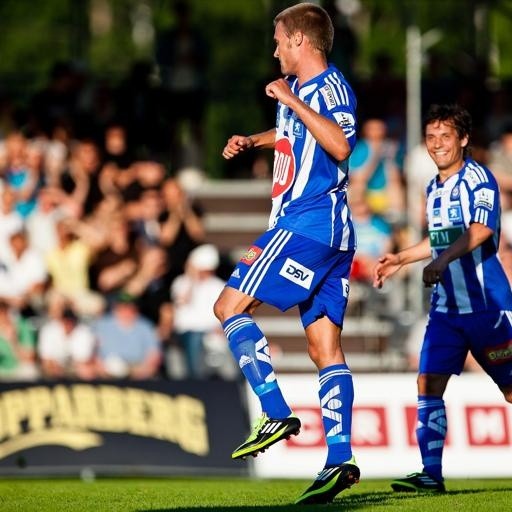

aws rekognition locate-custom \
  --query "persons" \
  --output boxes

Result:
[345,112,511,372]
[250,155,269,178]
[0,114,240,385]
[372,104,512,491]
[212,3,361,505]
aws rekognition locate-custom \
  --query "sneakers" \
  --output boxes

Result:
[294,455,360,506]
[231,411,301,462]
[391,472,446,493]
